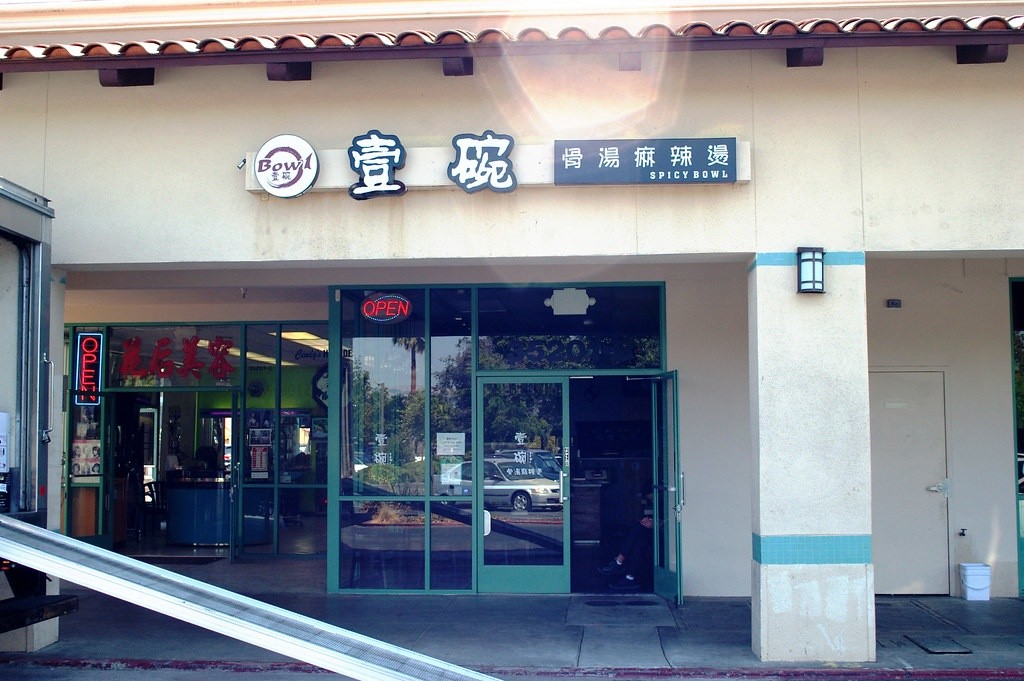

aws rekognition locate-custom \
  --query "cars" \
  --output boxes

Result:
[480,442,624,496]
[432,457,563,513]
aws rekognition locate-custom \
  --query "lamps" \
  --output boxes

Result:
[797,246,823,294]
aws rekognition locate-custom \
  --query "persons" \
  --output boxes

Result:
[72,445,101,475]
[597,474,663,591]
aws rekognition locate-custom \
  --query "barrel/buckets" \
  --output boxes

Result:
[959,563,991,601]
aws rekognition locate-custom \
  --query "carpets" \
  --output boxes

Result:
[563,597,677,627]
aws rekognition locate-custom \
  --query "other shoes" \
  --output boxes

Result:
[609,574,640,590]
[598,559,625,575]
